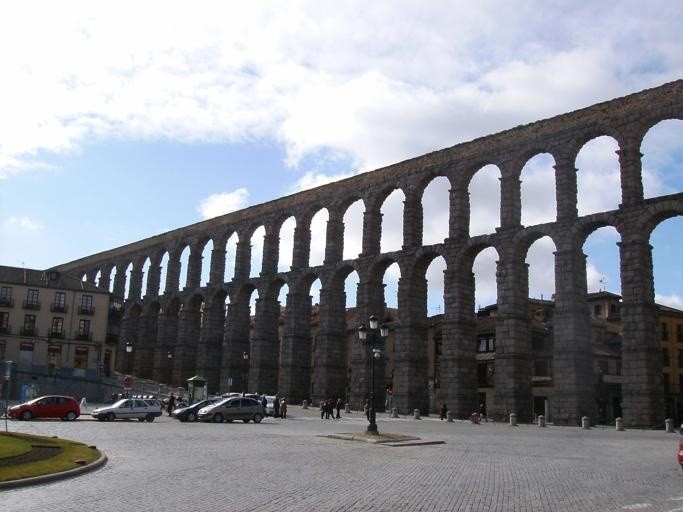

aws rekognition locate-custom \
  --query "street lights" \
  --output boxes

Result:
[243,352,249,390]
[358,314,388,435]
[167,351,172,387]
[126,342,134,374]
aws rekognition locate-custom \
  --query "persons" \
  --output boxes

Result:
[273,396,280,418]
[479,403,489,423]
[187,393,194,406]
[364,399,369,420]
[319,398,343,420]
[439,402,448,419]
[280,398,287,419]
[262,395,269,418]
[167,392,174,416]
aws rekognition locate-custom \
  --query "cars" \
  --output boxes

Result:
[172,392,282,424]
[9,396,80,421]
[91,398,163,422]
